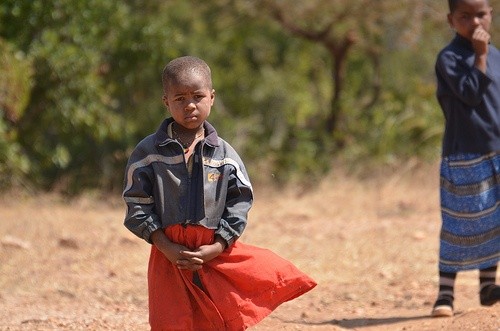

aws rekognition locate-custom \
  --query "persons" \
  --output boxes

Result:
[429,0,499,320]
[121,55,257,331]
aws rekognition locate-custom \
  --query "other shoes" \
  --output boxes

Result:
[433,297,455,316]
[479,285,500,306]
